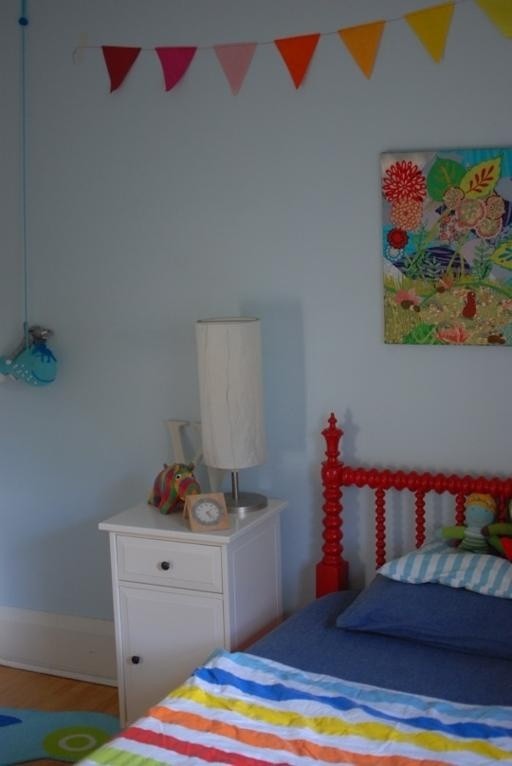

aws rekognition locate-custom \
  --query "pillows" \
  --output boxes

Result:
[335,542,512,660]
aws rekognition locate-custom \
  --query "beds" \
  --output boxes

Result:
[75,412,511,766]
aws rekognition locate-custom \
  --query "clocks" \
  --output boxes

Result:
[182,492,231,532]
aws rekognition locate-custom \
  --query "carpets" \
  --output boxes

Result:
[0,708,119,765]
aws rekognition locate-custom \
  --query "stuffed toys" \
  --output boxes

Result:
[440,493,497,557]
[479,496,511,563]
[146,460,202,515]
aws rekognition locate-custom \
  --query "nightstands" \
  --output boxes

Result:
[97,498,290,732]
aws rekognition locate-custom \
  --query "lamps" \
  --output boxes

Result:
[196,317,268,514]
